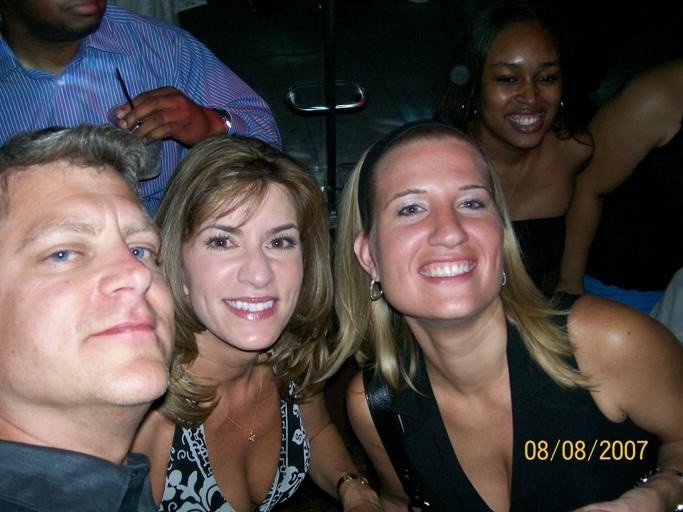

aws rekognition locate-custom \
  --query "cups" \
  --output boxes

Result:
[110,105,165,185]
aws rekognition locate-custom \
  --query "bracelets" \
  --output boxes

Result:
[335,471,369,493]
[634,466,682,489]
[212,107,232,132]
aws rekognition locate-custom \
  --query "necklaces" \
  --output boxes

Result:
[217,354,262,443]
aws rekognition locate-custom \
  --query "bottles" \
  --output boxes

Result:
[304,148,329,201]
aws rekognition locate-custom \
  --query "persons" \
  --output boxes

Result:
[0,123,178,512]
[309,118,683,512]
[555,59,683,317]
[127,132,387,510]
[430,3,597,299]
[0,1,281,226]
